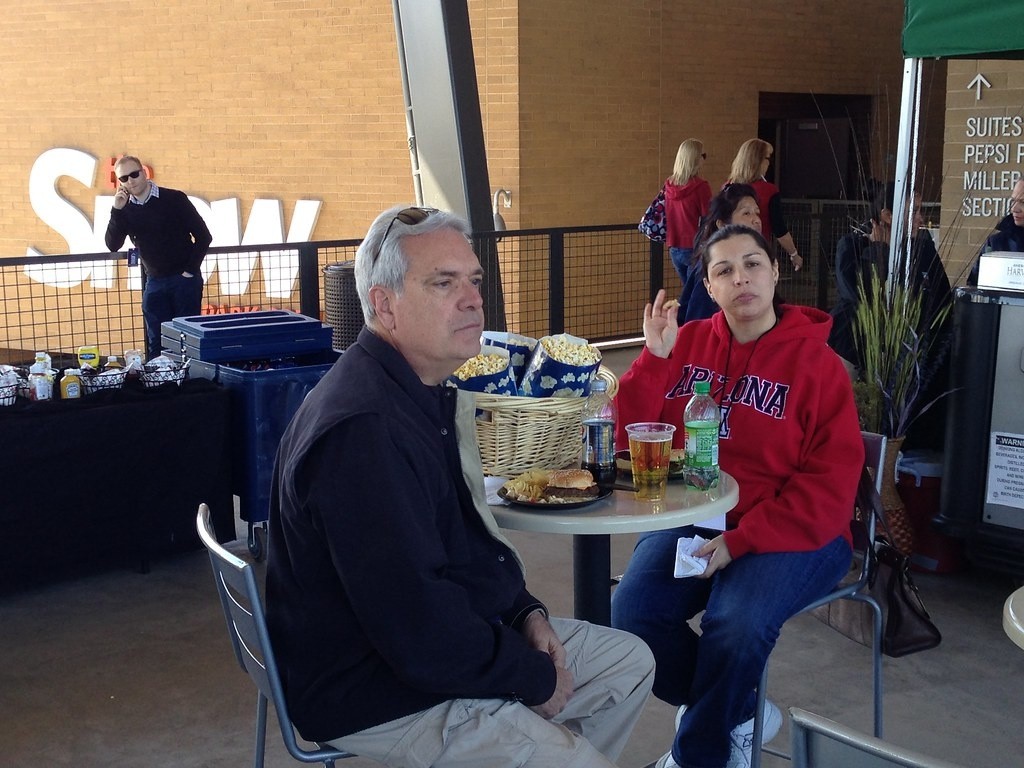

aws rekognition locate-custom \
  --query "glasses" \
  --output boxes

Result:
[371,207,439,269]
[118,170,141,183]
[701,153,707,160]
[764,156,773,162]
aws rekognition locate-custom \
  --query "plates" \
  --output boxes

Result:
[613,449,685,479]
[496,486,612,509]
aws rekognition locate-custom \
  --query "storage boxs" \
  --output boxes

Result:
[161,310,344,562]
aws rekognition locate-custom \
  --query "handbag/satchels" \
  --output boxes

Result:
[805,466,943,659]
[637,182,666,244]
[688,178,733,257]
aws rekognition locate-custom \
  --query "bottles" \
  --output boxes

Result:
[60,369,80,399]
[104,356,121,368]
[581,379,617,487]
[683,381,721,492]
[29,351,51,401]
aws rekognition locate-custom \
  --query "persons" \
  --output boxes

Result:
[677,183,762,327]
[263,205,656,768]
[966,176,1024,286]
[665,138,712,286]
[611,224,865,768]
[105,156,213,361]
[835,181,952,368]
[720,139,803,268]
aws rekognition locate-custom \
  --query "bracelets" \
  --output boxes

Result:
[789,249,797,256]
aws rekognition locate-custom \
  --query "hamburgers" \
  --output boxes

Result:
[543,468,600,502]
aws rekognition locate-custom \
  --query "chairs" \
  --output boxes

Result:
[197,502,360,767]
[750,434,944,768]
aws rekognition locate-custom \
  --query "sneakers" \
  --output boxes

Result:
[655,705,690,768]
[726,695,783,768]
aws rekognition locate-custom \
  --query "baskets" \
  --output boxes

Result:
[134,361,190,392]
[0,381,20,406]
[470,365,620,481]
[14,365,60,399]
[76,366,130,402]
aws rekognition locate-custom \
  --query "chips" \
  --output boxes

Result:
[502,467,557,502]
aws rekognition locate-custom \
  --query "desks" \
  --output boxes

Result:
[484,448,739,629]
[0,376,238,606]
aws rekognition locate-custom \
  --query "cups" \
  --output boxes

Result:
[626,422,676,501]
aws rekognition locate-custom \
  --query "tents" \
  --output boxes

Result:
[887,0,1024,308]
